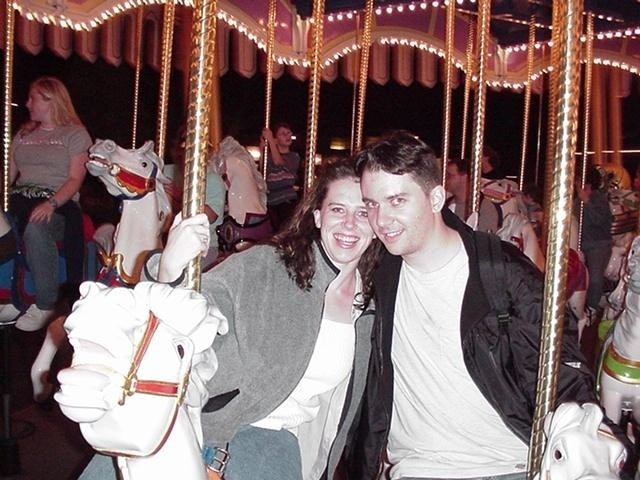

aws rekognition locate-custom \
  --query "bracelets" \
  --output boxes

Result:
[48,199,59,210]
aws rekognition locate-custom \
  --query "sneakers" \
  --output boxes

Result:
[0,302,23,326]
[16,304,58,332]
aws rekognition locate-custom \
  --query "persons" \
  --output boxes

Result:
[79,121,639,479]
[0,76,92,331]
